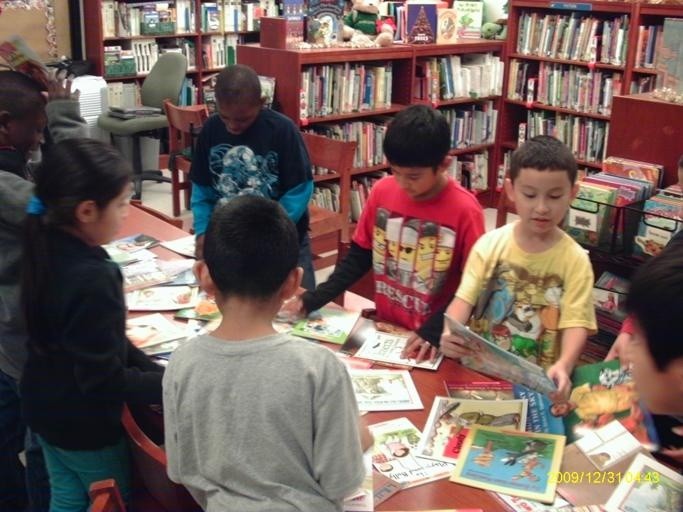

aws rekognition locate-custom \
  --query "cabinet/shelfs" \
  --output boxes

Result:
[606,90,682,188]
[231,40,510,255]
[84,0,339,170]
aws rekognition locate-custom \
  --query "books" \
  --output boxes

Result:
[99,0,683,366]
[101,233,683,511]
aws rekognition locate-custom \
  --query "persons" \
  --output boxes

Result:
[1,70,90,512]
[284,106,485,367]
[20,137,165,512]
[165,193,374,512]
[436,135,599,400]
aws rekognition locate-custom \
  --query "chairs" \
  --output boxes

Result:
[300,130,356,272]
[88,478,126,512]
[128,199,183,229]
[161,97,210,217]
[118,402,204,512]
[97,52,187,200]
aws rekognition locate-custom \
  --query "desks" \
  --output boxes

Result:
[100,201,556,512]
